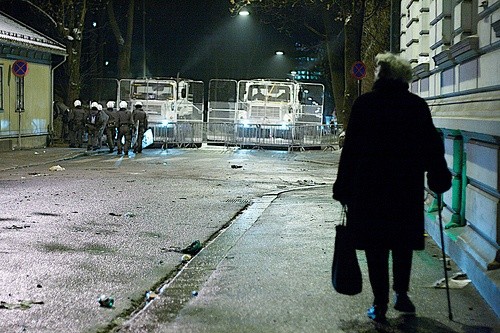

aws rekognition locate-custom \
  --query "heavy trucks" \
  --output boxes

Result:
[127,78,195,147]
[234,80,305,146]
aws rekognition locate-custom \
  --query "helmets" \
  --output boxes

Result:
[98,104,103,110]
[119,101,127,109]
[135,101,143,106]
[107,101,115,109]
[91,102,98,109]
[74,100,81,107]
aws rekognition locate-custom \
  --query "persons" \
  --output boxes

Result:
[53,99,148,155]
[332,52,453,321]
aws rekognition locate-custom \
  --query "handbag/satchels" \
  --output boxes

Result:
[331,205,363,297]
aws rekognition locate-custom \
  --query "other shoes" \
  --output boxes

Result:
[367,303,385,321]
[393,294,417,313]
